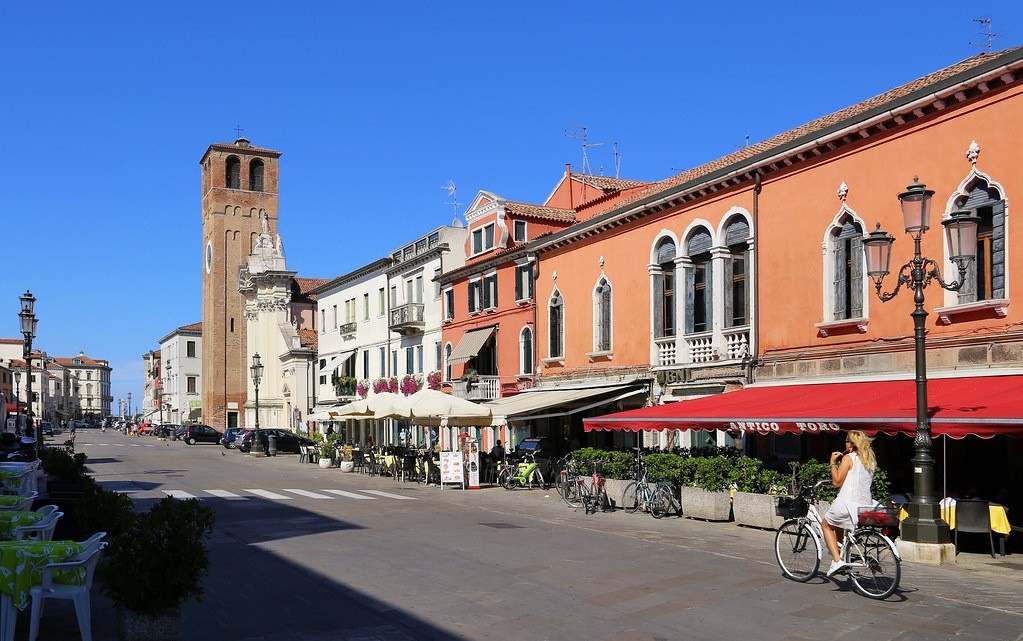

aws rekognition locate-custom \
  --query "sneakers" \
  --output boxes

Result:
[827,559,847,577]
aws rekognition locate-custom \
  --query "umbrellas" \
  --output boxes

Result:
[309,388,493,458]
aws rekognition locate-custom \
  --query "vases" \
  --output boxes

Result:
[434,372,441,382]
[341,461,354,473]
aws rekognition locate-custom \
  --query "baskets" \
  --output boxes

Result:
[772,492,808,517]
[857,506,900,528]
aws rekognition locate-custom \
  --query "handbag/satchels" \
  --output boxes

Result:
[778,495,808,517]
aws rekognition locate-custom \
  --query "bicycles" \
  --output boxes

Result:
[499,448,546,491]
[774,479,901,600]
[554,452,607,514]
[622,465,673,519]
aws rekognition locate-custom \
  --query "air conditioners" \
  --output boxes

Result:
[394,306,418,325]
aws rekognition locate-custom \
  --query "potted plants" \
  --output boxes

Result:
[94,496,218,641]
[460,365,479,383]
[64,484,137,575]
[316,440,336,469]
[571,444,891,546]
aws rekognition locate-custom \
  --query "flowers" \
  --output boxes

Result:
[356,377,398,396]
[400,375,419,395]
[426,370,441,390]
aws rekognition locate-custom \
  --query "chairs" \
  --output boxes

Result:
[14,495,39,511]
[906,493,913,501]
[954,498,996,560]
[16,512,64,541]
[28,505,58,538]
[20,491,38,499]
[40,531,107,618]
[25,457,42,491]
[28,542,109,641]
[298,442,554,488]
[999,524,1023,556]
[892,495,907,502]
[0,469,33,496]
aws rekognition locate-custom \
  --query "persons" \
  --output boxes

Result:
[491,439,504,462]
[326,425,337,434]
[432,444,442,460]
[822,430,877,576]
[59,417,152,440]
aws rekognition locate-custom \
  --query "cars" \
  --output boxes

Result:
[153,424,180,437]
[74,420,90,428]
[113,421,128,430]
[235,427,315,455]
[219,427,244,449]
[139,423,157,435]
[175,425,188,440]
[40,423,53,437]
[184,425,224,445]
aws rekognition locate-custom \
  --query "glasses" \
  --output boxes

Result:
[843,440,853,445]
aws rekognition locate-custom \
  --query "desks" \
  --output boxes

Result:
[892,497,1012,557]
[364,454,383,475]
[422,460,447,485]
[0,462,86,641]
[378,455,400,477]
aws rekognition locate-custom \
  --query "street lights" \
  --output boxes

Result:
[128,392,131,423]
[156,380,165,438]
[861,173,983,543]
[14,371,22,436]
[249,352,264,451]
[18,289,39,438]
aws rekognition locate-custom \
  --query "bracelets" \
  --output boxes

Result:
[829,464,838,469]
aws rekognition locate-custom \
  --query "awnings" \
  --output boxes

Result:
[480,383,640,427]
[6,403,24,411]
[320,350,355,376]
[447,326,495,366]
[582,367,1023,438]
